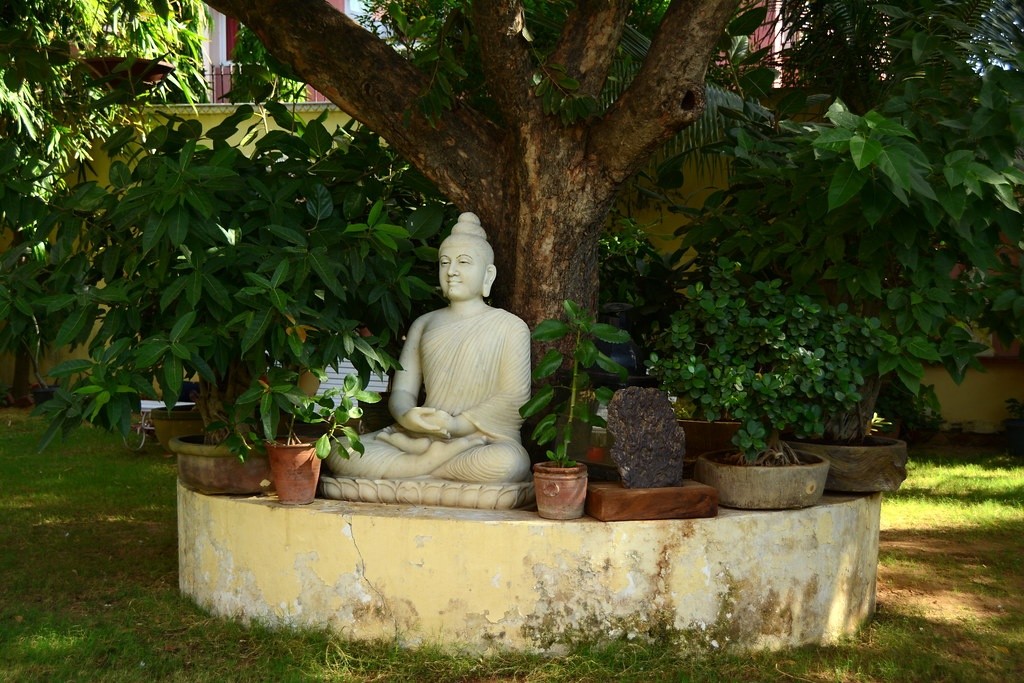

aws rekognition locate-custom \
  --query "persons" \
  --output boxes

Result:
[324,212,532,485]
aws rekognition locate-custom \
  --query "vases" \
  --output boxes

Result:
[676,420,742,460]
[150,405,204,457]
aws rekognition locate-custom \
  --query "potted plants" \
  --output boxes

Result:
[0,63,449,497]
[203,365,383,506]
[667,63,1024,495]
[643,258,902,509]
[518,299,630,521]
